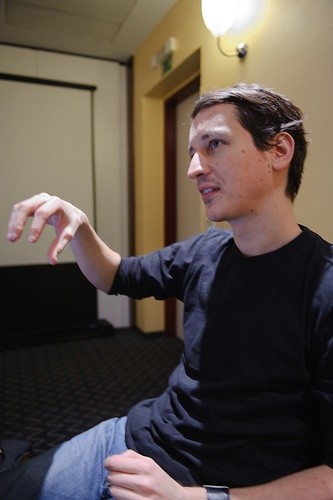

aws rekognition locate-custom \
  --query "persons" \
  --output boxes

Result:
[1,82,332,500]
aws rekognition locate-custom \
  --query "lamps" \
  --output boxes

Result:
[200,0,247,58]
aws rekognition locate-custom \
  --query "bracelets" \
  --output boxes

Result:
[202,484,231,500]
